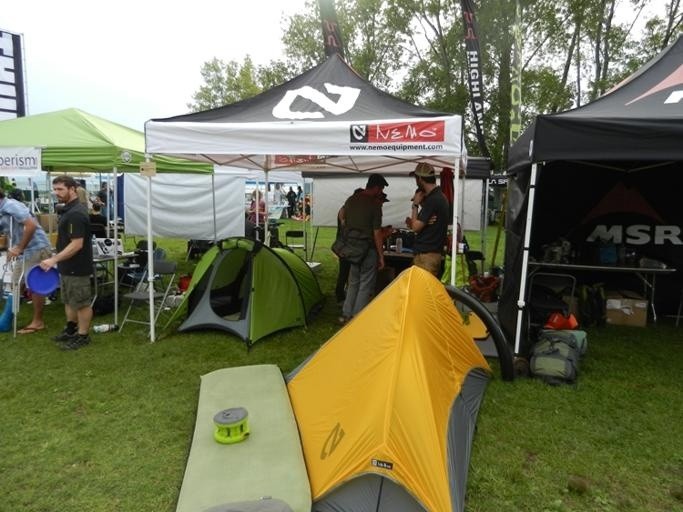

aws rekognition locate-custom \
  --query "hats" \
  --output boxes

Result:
[368,173,388,187]
[408,162,434,178]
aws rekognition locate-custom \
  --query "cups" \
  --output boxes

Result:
[459,243,463,252]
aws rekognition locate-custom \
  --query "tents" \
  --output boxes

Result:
[153,236,328,354]
[282,266,498,512]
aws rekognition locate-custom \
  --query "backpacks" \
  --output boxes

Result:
[530,327,588,386]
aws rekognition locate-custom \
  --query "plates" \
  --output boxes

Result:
[27,265,59,293]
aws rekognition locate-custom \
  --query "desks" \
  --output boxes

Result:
[383,251,416,279]
[91,254,138,309]
[527,258,677,326]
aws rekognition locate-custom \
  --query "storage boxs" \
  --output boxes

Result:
[602,289,650,328]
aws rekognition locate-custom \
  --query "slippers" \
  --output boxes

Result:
[16,325,46,335]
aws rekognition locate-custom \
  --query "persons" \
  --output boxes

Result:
[295,186,302,201]
[0,174,112,351]
[248,190,265,223]
[285,184,298,218]
[336,162,451,328]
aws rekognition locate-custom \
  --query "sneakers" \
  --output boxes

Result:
[59,335,91,350]
[50,330,78,342]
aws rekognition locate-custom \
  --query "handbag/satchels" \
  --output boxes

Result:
[330,237,367,265]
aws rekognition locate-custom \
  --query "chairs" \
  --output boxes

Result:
[285,230,305,254]
[115,239,179,340]
[527,272,577,339]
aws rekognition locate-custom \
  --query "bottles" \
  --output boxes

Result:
[395,235,403,254]
